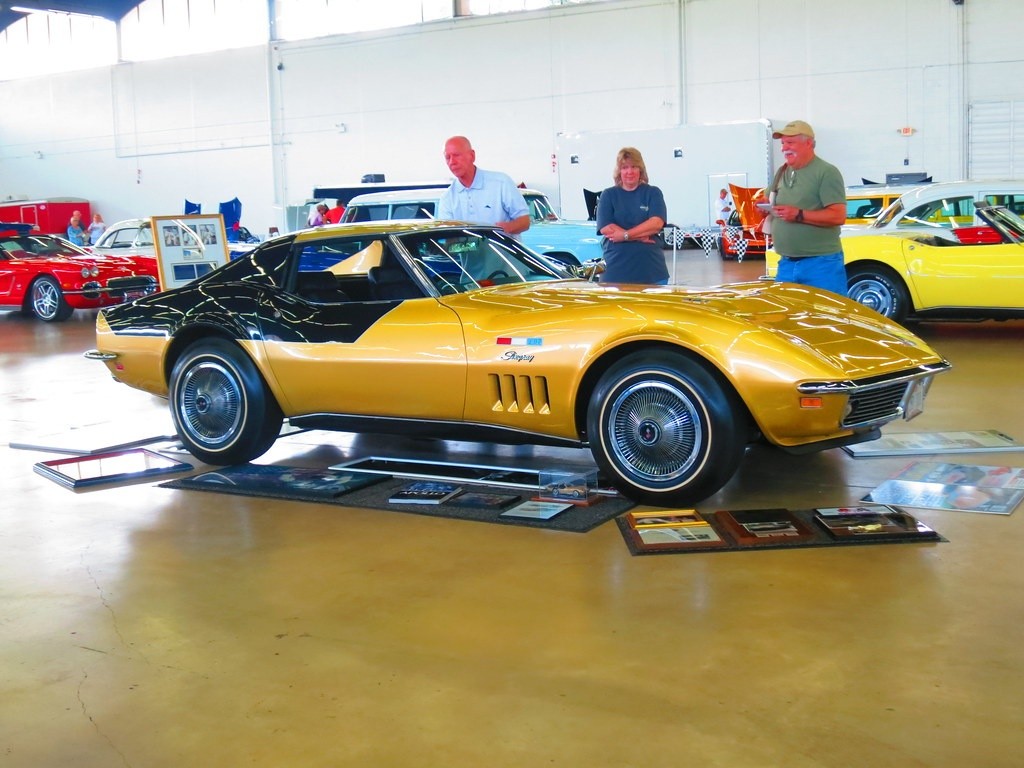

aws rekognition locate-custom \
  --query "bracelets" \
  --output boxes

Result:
[624,231,628,241]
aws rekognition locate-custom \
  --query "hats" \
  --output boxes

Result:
[772,120,814,139]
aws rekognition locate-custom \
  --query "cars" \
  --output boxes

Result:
[79,220,157,258]
[338,188,604,279]
[0,223,157,322]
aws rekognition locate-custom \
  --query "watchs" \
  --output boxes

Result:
[795,209,804,222]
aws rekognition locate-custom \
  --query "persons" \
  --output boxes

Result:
[322,199,345,224]
[66,211,86,246]
[715,189,731,231]
[184,229,197,245]
[312,204,326,226]
[753,121,848,297]
[87,214,106,246]
[202,225,213,244]
[596,147,670,285]
[438,136,530,285]
[165,227,180,245]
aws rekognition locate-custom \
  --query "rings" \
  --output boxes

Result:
[780,215,783,218]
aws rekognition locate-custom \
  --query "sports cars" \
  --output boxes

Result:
[80,219,949,507]
[839,185,997,224]
[765,180,1024,324]
[716,210,773,260]
